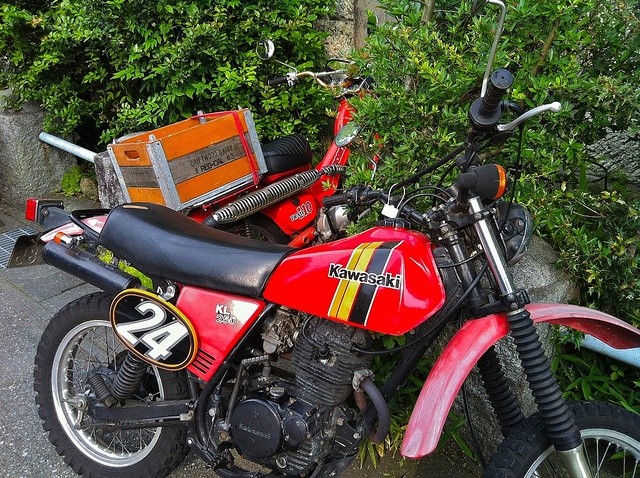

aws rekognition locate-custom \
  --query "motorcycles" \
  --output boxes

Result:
[105,36,387,251]
[6,0,640,477]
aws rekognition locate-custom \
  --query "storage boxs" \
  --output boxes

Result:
[107,105,269,214]
